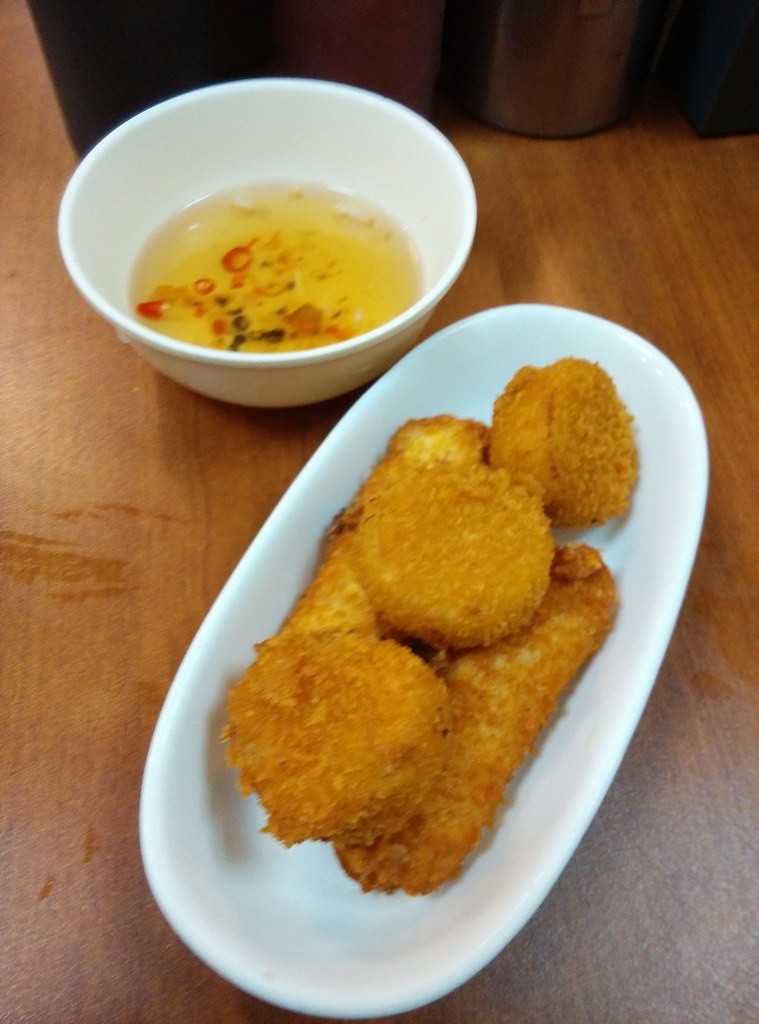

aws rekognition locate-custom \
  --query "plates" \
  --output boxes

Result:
[144,301,709,1019]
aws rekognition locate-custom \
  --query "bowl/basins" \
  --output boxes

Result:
[56,77,477,409]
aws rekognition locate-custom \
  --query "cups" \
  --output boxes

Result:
[16,0,759,160]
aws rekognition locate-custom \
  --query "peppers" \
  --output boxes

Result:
[136,237,257,336]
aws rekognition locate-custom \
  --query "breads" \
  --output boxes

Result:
[216,357,641,899]
